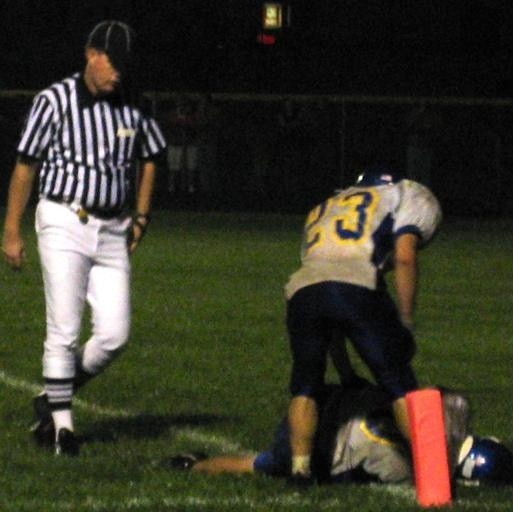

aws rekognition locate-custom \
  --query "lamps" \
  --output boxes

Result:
[262,0,282,29]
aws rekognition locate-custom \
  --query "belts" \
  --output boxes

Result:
[38,191,126,219]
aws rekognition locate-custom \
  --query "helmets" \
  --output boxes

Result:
[454,432,513,487]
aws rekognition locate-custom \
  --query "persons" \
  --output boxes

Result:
[166,374,510,491]
[1,19,168,459]
[283,165,445,490]
[0,0,512,216]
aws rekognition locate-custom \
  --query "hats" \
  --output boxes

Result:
[355,171,393,187]
[87,20,138,69]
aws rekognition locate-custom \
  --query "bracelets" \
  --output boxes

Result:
[400,319,418,333]
[132,218,147,236]
[134,211,151,224]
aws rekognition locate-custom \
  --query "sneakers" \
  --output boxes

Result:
[52,427,80,461]
[286,465,320,490]
[28,389,55,447]
